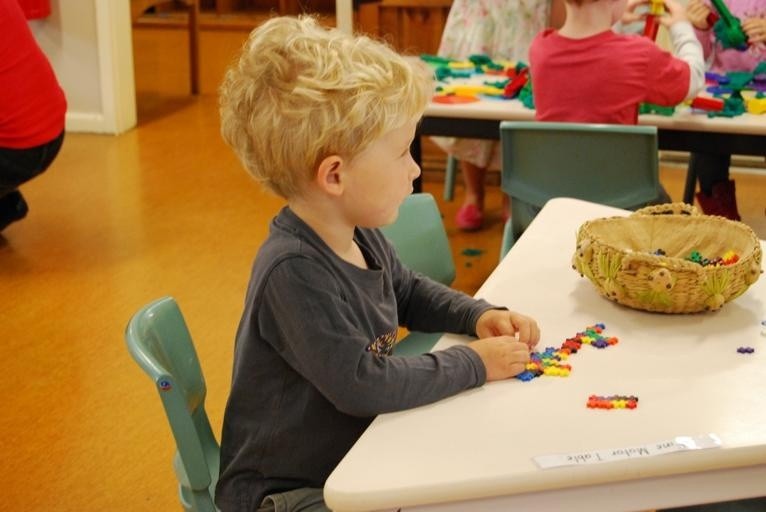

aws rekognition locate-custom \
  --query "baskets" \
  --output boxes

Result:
[570,201,765,315]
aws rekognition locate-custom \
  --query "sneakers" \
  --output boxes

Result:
[0,189,28,230]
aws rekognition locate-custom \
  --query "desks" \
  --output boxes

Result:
[403,55,766,216]
[322,197,766,512]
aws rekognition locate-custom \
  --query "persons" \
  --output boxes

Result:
[213,18,541,512]
[436,0,548,230]
[532,1,705,215]
[1,0,66,232]
[686,0,765,224]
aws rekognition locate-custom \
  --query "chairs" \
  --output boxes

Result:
[124,296,225,512]
[378,193,456,356]
[500,120,673,259]
